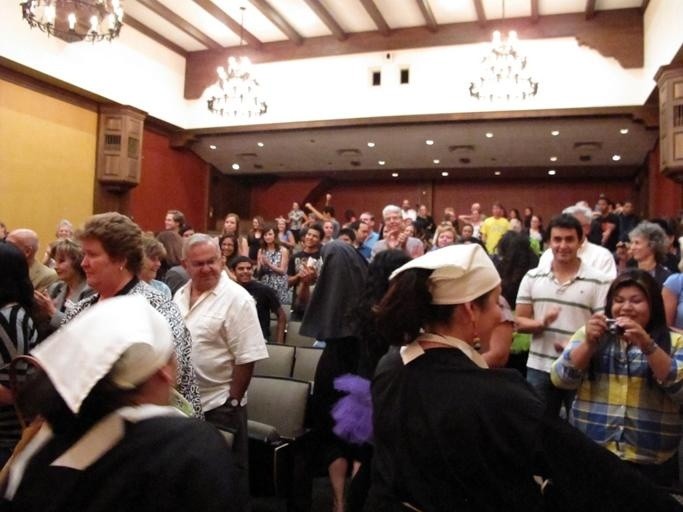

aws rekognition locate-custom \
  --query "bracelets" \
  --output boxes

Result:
[641,339,659,357]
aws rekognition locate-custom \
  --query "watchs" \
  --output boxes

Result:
[222,397,247,416]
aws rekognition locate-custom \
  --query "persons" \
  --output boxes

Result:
[368,240,682,512]
[0,219,94,326]
[310,252,417,509]
[171,233,268,475]
[6,296,250,510]
[515,217,610,417]
[139,195,683,348]
[0,239,41,450]
[58,210,206,419]
[550,271,682,485]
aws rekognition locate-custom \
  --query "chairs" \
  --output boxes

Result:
[245,376,311,482]
[292,345,324,383]
[269,304,292,319]
[269,319,279,343]
[286,320,317,347]
[253,342,296,379]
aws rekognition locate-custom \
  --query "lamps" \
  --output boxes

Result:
[18,0,125,43]
[207,55,268,119]
[469,30,539,103]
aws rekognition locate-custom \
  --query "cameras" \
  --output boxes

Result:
[606,317,625,337]
